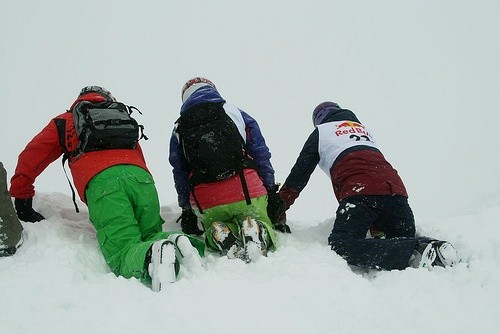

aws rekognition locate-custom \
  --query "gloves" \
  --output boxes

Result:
[175,209,203,234]
[13,198,45,223]
[274,223,291,233]
[266,184,284,223]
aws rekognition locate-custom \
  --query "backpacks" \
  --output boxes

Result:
[72,99,139,152]
[177,101,250,182]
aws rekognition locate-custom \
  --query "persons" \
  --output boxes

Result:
[279,102,464,271]
[169,77,287,263]
[0,162,24,257]
[10,86,207,292]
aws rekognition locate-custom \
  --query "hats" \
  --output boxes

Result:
[313,101,340,127]
[182,77,217,103]
[79,85,115,103]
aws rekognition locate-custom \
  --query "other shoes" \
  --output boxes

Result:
[410,243,437,269]
[150,239,177,292]
[241,216,267,263]
[432,240,460,269]
[210,220,250,263]
[168,234,201,270]
